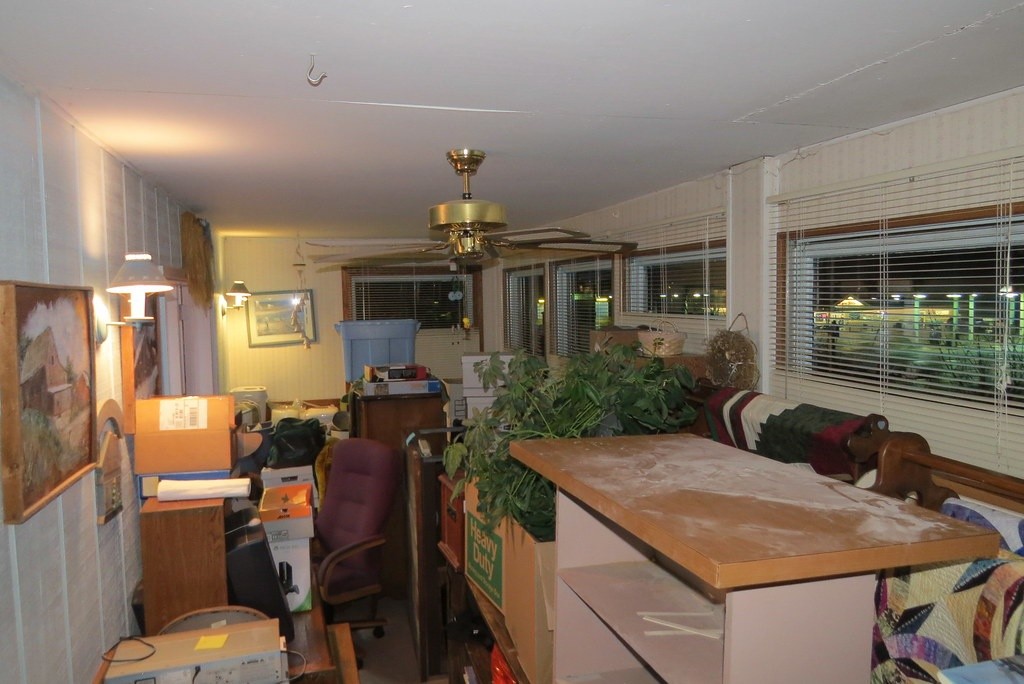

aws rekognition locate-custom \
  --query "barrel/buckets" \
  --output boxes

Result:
[228,386,267,422]
[247,421,275,451]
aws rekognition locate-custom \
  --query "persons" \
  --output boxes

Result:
[830,318,963,347]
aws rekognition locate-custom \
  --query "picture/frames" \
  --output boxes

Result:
[244,289,318,348]
[118,292,163,435]
[0,280,100,523]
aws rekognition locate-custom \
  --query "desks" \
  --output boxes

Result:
[288,560,361,684]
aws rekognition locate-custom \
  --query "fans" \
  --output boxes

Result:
[307,148,639,266]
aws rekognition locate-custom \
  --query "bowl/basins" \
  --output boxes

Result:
[332,410,349,431]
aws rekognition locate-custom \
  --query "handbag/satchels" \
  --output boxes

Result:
[268,417,326,463]
[637,319,687,355]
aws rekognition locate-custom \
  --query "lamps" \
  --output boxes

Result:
[94,250,175,350]
[218,280,251,317]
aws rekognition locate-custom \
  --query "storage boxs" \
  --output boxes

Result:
[463,475,555,684]
[333,318,517,425]
[258,466,318,612]
[134,394,239,474]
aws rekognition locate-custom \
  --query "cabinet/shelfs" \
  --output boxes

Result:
[351,388,448,599]
[510,432,1002,684]
[405,440,448,684]
[446,560,530,684]
[139,496,231,635]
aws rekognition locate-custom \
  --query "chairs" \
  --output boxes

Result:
[310,437,399,668]
